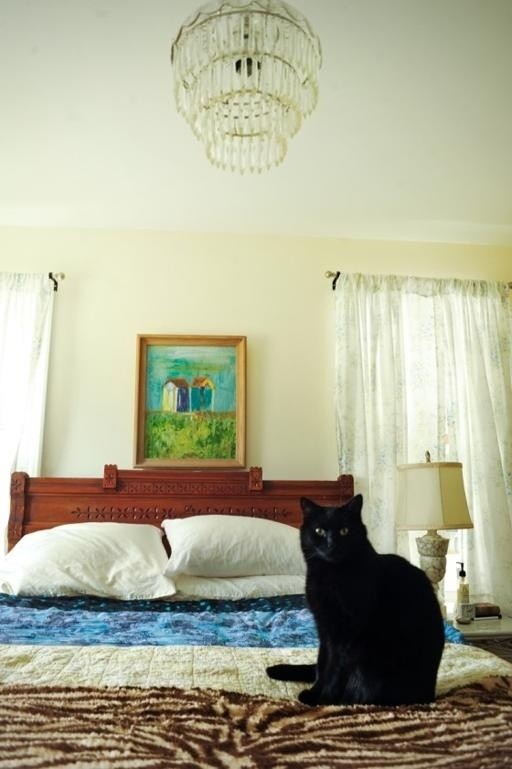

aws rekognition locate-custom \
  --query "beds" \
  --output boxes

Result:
[0,464,512,769]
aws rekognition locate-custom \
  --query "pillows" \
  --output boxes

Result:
[0,521,178,600]
[160,514,306,578]
[164,575,308,602]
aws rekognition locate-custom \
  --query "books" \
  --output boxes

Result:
[472,616,499,621]
[470,601,501,617]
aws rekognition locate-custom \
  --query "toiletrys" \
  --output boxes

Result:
[457,559,474,625]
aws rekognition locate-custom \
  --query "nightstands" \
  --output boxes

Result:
[451,617,512,663]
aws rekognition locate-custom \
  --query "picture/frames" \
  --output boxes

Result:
[133,334,247,471]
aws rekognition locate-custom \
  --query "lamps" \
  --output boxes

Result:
[394,450,474,595]
[170,0,323,173]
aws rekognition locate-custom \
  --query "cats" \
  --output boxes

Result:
[265,493,445,708]
